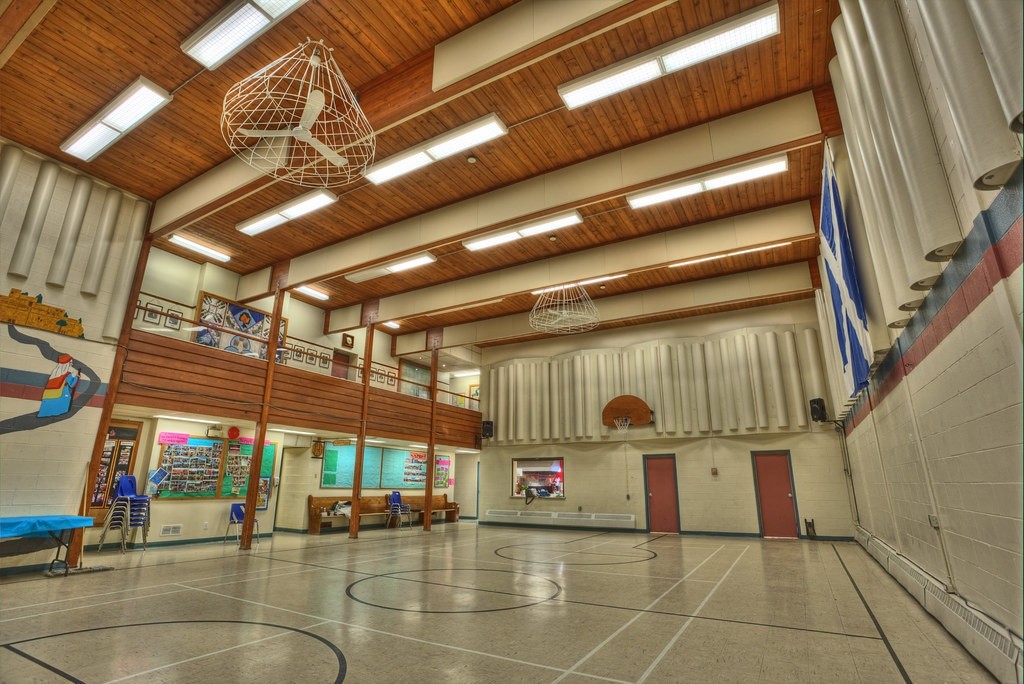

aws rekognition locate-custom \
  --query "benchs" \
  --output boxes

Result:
[307,495,460,535]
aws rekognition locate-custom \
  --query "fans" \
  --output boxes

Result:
[237,49,349,167]
[548,288,586,324]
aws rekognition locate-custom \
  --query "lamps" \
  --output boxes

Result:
[360,113,509,185]
[166,231,231,263]
[345,250,438,284]
[557,1,781,111]
[59,77,175,163]
[294,286,330,301]
[462,209,584,252]
[235,188,340,237]
[179,0,308,72]
[626,152,788,210]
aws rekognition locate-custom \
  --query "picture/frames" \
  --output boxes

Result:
[293,345,305,362]
[164,309,183,330]
[134,299,141,319]
[387,372,397,386]
[357,364,364,376]
[319,352,330,369]
[306,348,317,365]
[371,368,377,381]
[143,301,163,324]
[377,369,386,382]
[284,342,293,359]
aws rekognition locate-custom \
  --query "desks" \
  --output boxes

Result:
[0,516,94,577]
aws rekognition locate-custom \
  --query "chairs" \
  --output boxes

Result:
[224,503,260,546]
[98,475,151,555]
[388,491,413,530]
